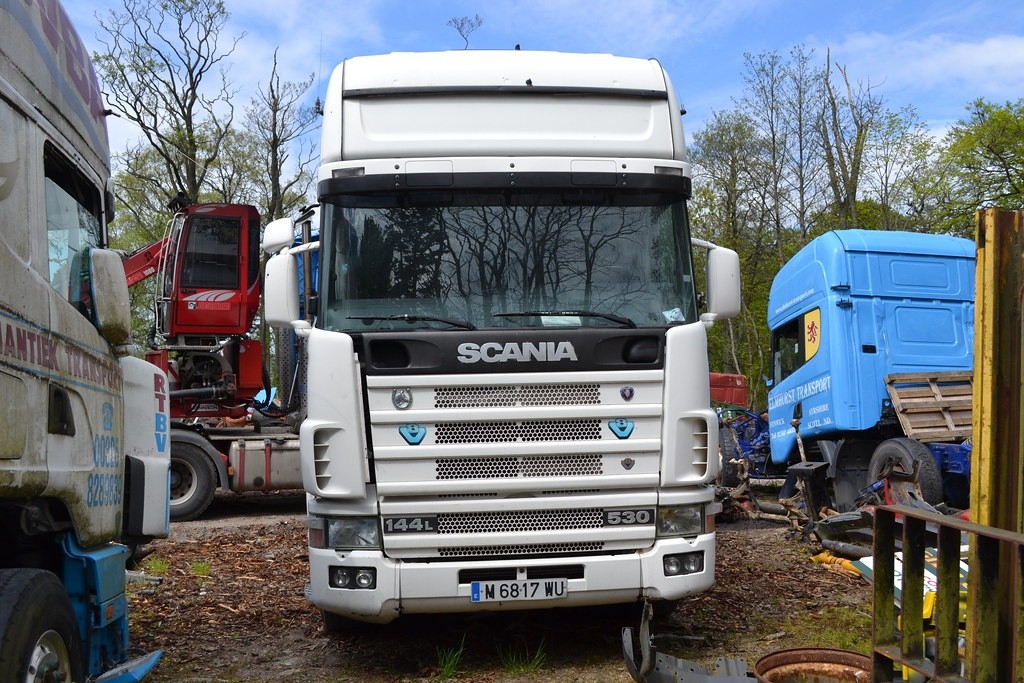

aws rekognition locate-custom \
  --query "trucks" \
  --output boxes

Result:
[261,47,748,635]
[0,0,136,683]
[765,227,976,560]
[140,193,304,521]
